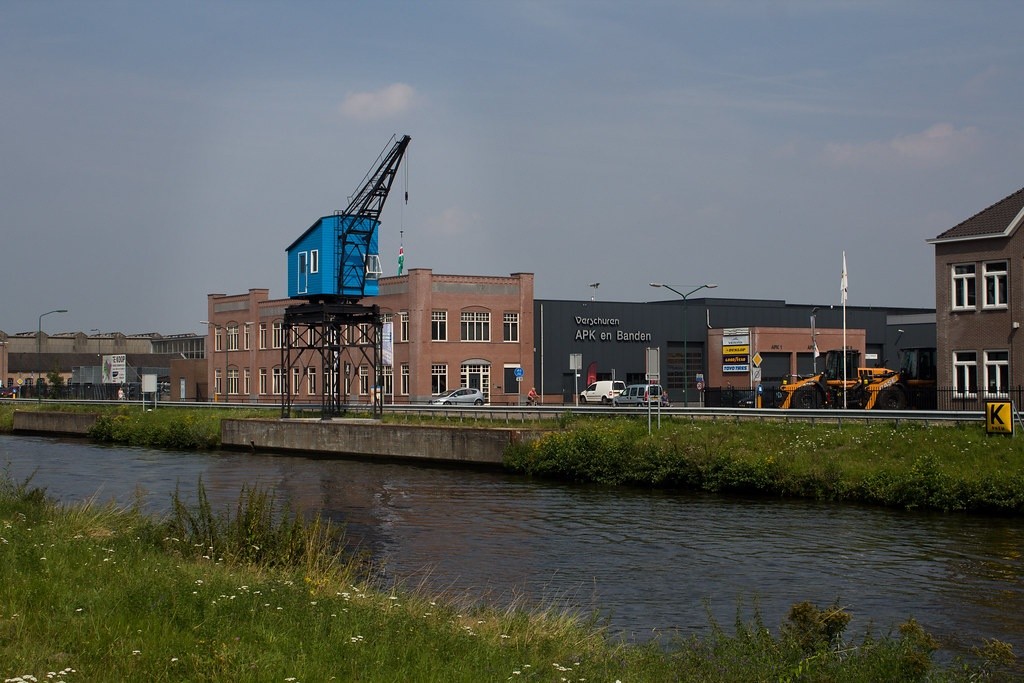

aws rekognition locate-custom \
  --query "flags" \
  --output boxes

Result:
[398,243,404,281]
[840,256,848,303]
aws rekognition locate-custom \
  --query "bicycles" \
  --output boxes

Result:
[118,393,126,400]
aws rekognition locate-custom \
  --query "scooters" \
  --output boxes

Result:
[526,396,539,406]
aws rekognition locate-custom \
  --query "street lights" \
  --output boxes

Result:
[650,282,719,407]
[199,320,229,402]
[38,309,68,404]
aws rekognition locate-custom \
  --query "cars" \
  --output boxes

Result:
[427,387,484,405]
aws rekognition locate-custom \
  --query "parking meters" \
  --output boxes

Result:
[757,384,763,408]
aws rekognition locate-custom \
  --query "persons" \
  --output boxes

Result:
[527,387,539,403]
[660,391,671,406]
[118,387,124,401]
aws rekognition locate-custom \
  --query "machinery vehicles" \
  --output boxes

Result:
[775,349,896,410]
[863,347,937,410]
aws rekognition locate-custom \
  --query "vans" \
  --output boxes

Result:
[580,380,627,404]
[612,384,664,407]
